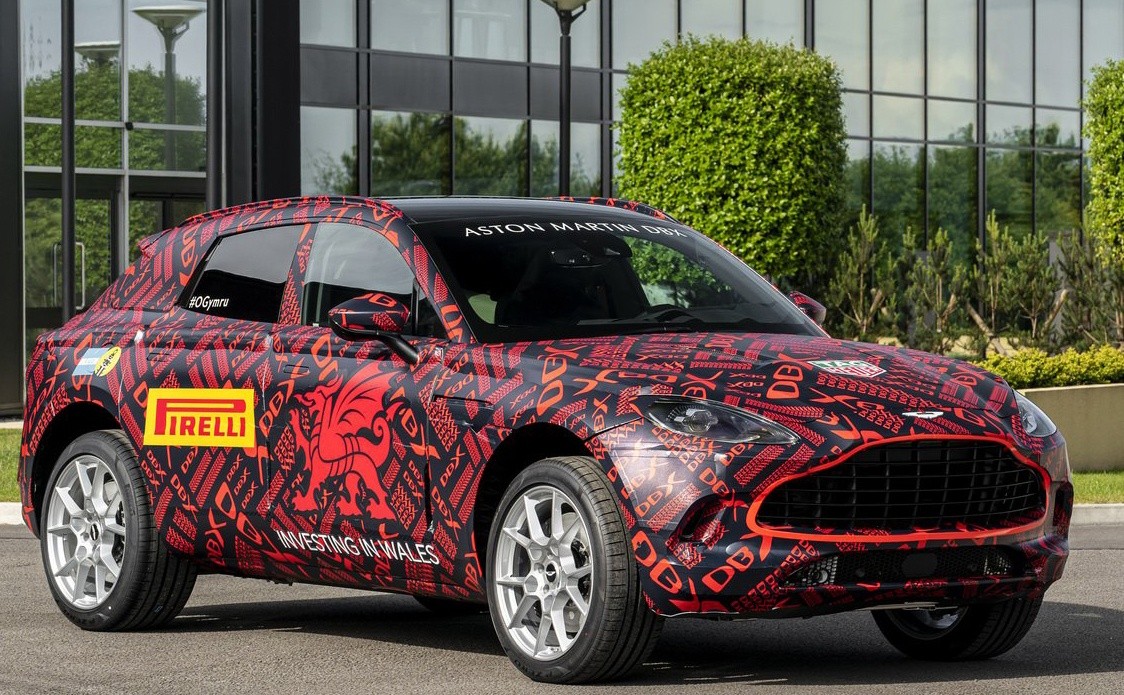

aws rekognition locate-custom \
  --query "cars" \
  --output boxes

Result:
[16,195,1076,684]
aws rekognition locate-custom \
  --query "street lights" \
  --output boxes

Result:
[132,7,208,236]
[544,1,592,193]
